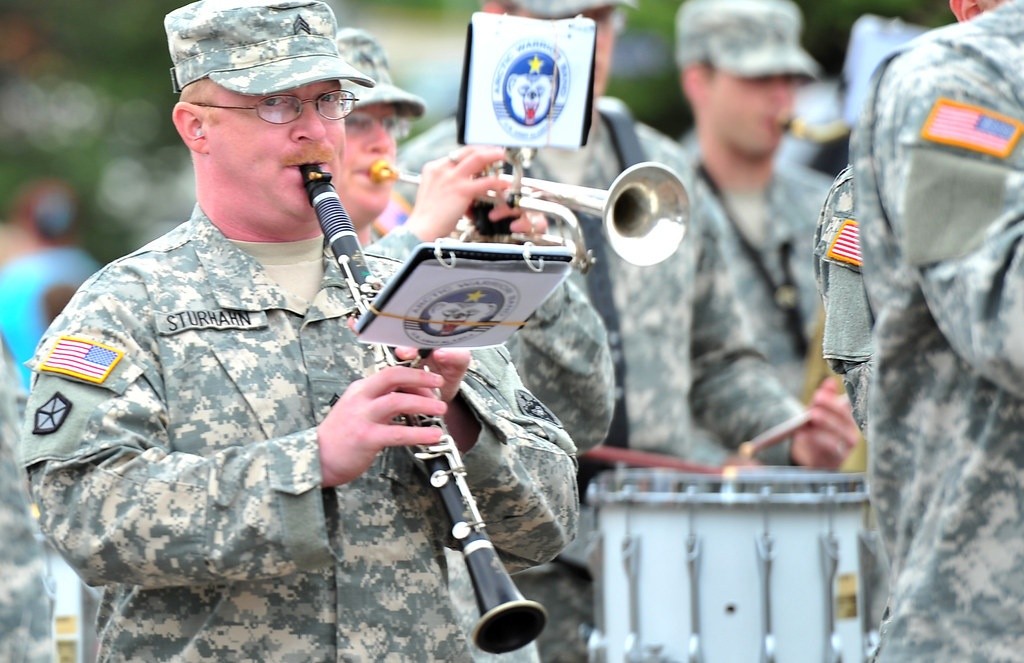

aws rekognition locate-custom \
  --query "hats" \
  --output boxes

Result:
[511,0,639,18]
[163,0,375,96]
[333,28,426,120]
[676,1,822,83]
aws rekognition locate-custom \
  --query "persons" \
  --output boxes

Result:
[0,0,1024,663]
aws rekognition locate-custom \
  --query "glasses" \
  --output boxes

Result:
[593,10,627,43]
[188,89,359,124]
[342,112,411,139]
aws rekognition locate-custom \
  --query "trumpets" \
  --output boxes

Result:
[370,144,693,270]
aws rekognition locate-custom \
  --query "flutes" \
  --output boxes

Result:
[298,162,550,655]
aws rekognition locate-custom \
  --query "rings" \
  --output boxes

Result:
[449,152,459,164]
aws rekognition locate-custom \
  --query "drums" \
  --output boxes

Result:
[589,467,875,663]
